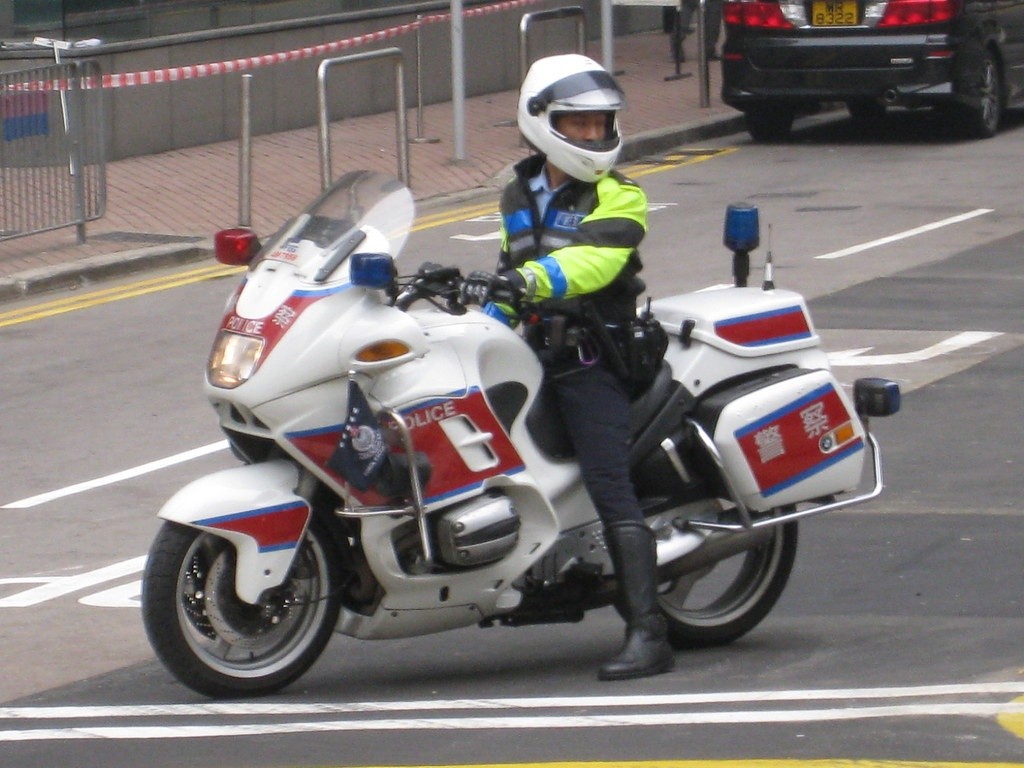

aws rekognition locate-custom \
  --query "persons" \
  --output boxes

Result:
[671,0,726,63]
[459,53,679,682]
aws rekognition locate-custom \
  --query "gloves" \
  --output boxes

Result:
[462,271,515,305]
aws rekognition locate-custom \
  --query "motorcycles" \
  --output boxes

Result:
[141,168,907,705]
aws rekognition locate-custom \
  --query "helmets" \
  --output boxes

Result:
[518,54,623,183]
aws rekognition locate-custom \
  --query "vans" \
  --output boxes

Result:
[720,1,1023,146]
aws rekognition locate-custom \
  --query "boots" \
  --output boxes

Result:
[596,521,676,680]
